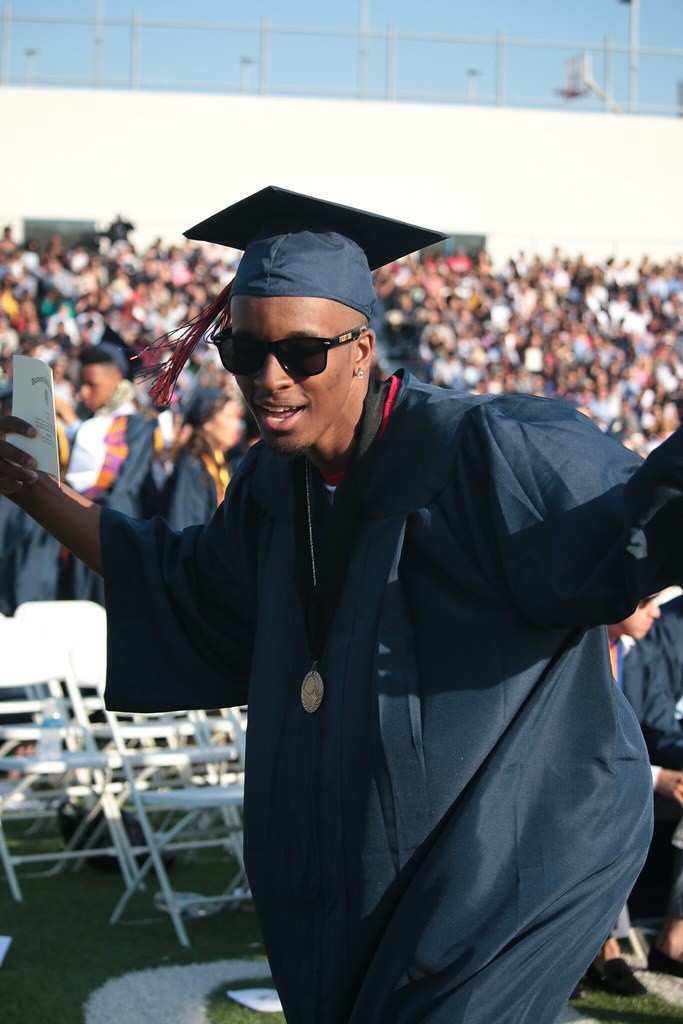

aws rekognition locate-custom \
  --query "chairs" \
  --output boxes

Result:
[0,599,254,948]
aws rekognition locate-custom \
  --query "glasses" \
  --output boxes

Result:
[211,326,367,377]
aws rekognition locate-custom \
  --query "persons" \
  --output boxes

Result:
[0,184,683,1024]
[0,218,683,726]
[590,586,683,999]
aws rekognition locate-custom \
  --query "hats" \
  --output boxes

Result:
[180,384,224,432]
[79,345,129,375]
[130,184,450,408]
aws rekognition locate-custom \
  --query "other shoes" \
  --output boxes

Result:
[648,945,683,977]
[586,958,647,997]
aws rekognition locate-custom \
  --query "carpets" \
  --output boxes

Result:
[80,954,683,1024]
